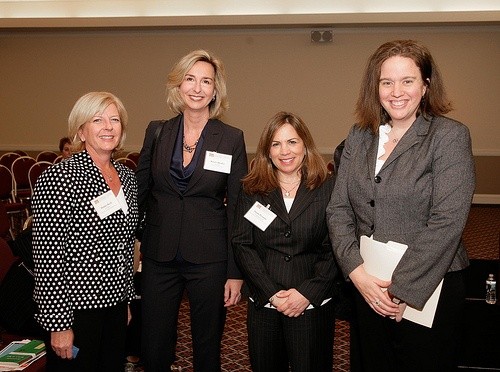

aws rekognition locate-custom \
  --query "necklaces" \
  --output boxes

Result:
[392,124,408,143]
[94,158,115,182]
[282,180,301,197]
[182,137,201,153]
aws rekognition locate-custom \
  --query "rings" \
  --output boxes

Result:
[375,300,381,306]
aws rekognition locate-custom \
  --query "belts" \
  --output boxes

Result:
[249,295,332,311]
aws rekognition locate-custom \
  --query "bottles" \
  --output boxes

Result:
[486,274,496,304]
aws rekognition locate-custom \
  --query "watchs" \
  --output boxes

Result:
[386,289,406,305]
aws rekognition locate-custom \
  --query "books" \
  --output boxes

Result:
[0,340,46,371]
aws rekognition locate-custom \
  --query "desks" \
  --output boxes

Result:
[351,259,500,372]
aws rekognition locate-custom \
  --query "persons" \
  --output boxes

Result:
[320,41,474,372]
[232,111,338,372]
[33,91,138,372]
[135,50,247,372]
[59,136,71,158]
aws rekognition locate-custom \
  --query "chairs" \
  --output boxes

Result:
[0,150,140,372]
